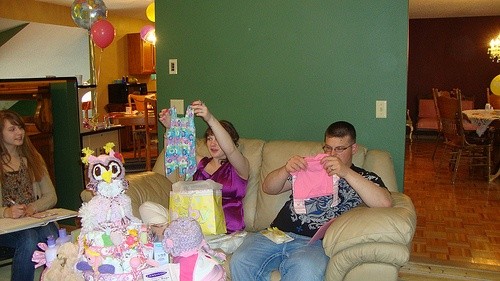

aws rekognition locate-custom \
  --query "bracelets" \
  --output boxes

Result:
[163,132,167,139]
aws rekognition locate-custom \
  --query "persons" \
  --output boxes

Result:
[228,121,393,281]
[0,111,62,281]
[139,99,249,255]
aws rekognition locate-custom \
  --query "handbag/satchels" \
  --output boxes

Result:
[168,188,228,235]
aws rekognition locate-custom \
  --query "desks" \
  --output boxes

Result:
[462,109,500,182]
[0,207,78,235]
[79,117,156,138]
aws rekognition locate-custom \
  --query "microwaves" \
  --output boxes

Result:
[108,83,147,104]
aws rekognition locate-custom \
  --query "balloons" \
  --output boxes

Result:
[90,20,114,49]
[490,74,500,96]
[145,3,156,23]
[139,25,155,45]
[70,0,108,30]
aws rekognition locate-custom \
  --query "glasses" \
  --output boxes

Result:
[322,143,355,153]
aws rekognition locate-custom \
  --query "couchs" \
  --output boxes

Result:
[81,139,416,281]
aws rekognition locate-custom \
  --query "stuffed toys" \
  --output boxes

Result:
[80,141,129,199]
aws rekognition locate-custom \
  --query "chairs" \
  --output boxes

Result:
[429,87,500,184]
[127,93,157,170]
[406,109,414,143]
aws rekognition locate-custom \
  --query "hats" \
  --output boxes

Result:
[162,216,206,258]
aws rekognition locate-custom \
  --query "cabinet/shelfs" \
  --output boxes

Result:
[127,33,156,75]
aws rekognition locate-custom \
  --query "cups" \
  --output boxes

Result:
[75,75,82,86]
[485,104,490,113]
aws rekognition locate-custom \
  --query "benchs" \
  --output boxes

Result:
[416,98,475,136]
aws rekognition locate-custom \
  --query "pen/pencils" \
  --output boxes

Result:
[7,197,16,205]
[146,272,167,278]
